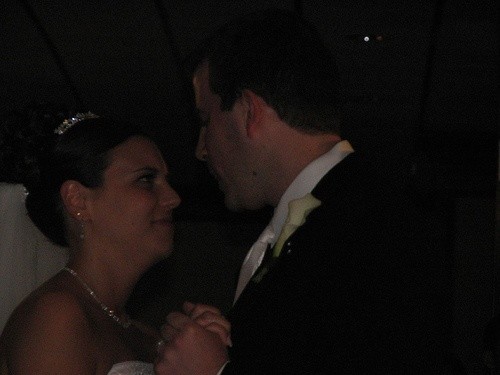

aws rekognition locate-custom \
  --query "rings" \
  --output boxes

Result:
[155,338,165,354]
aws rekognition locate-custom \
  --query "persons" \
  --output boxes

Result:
[0,102,205,375]
[145,23,473,375]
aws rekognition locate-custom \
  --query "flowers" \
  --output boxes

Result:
[251,192,321,286]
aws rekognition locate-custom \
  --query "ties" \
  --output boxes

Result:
[232,224,276,307]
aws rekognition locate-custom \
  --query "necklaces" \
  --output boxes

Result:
[64,263,152,337]
